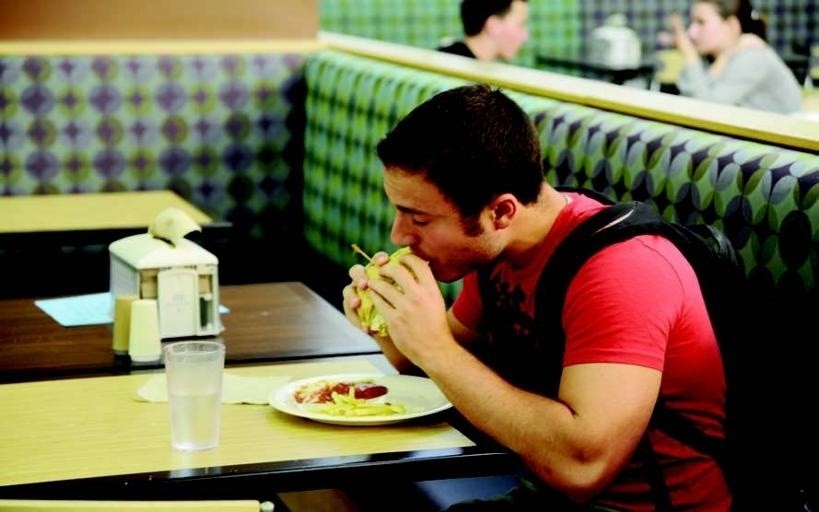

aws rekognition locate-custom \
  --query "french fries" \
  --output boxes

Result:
[309,384,405,417]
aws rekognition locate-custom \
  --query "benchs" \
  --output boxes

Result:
[242,25,818,512]
[318,0,819,97]
[1,30,326,299]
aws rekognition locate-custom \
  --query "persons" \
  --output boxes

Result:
[669,0,804,119]
[341,82,741,511]
[437,1,528,66]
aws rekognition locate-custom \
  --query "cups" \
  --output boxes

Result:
[167,447,224,480]
[165,340,226,453]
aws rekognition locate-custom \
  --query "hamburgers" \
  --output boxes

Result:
[354,244,428,339]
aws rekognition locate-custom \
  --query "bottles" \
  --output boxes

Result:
[130,301,164,363]
[113,294,136,358]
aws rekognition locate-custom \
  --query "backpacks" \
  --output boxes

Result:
[474,183,775,495]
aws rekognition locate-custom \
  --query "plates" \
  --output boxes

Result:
[266,370,453,427]
